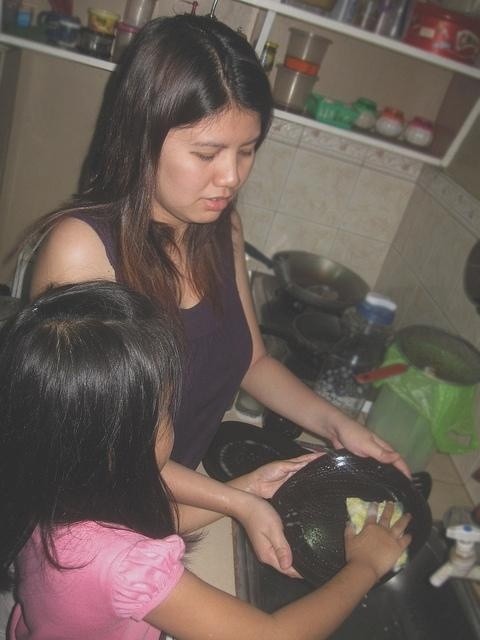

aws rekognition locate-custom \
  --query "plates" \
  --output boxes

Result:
[201,420,304,482]
[399,324,480,386]
[264,454,433,593]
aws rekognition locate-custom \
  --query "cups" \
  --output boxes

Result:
[57,0,157,61]
[333,0,412,40]
[271,27,334,114]
[366,383,438,475]
[235,335,287,419]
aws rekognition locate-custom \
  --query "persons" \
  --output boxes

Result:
[21,12,410,581]
[0,278,414,640]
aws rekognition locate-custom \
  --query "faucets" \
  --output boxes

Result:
[429,524,480,588]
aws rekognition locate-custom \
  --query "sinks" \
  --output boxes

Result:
[257,526,480,640]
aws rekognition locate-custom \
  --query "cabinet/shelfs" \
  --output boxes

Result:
[0,1,479,169]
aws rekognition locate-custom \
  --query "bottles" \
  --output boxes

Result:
[319,291,396,423]
[351,96,435,148]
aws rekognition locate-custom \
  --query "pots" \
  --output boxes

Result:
[243,240,368,311]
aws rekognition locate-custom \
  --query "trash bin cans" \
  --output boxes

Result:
[365,324,479,472]
